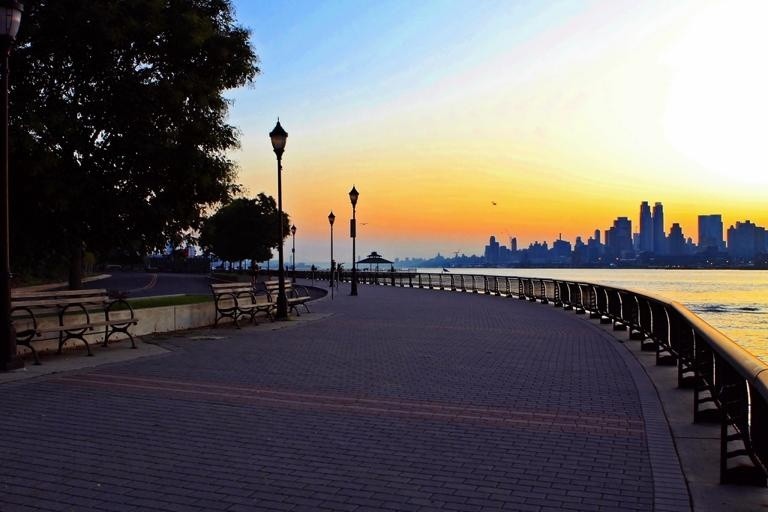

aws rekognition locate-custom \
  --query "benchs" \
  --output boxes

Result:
[211,278,311,328]
[10,289,138,365]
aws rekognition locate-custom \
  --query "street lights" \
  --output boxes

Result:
[349,185,360,296]
[290,223,297,275]
[270,121,288,324]
[328,212,336,288]
